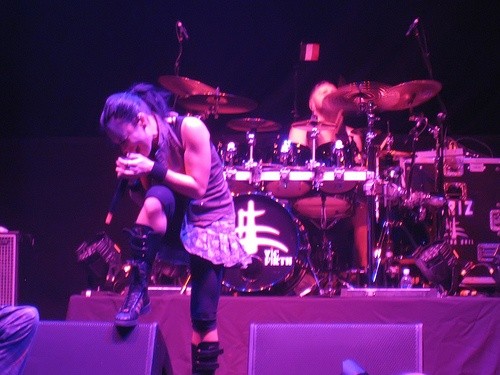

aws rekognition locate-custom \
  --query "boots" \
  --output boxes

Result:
[115,227,158,325]
[191,341,220,375]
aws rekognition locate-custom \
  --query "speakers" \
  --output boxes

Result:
[16,320,174,375]
[247,322,425,375]
[0,231,18,308]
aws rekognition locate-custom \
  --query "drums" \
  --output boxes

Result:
[219,191,311,296]
[316,140,362,195]
[210,136,263,193]
[262,137,314,198]
[289,187,357,221]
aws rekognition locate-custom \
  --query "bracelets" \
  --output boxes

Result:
[127,179,142,193]
[150,160,167,183]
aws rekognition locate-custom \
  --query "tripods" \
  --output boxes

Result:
[373,163,418,286]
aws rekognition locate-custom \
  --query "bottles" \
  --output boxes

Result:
[401,268,411,289]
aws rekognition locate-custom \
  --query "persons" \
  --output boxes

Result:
[288,81,369,273]
[99,85,238,375]
[0,304,39,375]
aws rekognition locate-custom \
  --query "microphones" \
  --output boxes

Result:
[406,18,419,36]
[105,176,127,225]
[177,21,190,40]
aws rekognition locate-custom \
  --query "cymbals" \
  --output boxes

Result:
[347,127,384,136]
[178,91,257,115]
[158,73,223,97]
[373,150,417,160]
[289,119,338,130]
[376,79,444,112]
[322,81,387,113]
[226,116,280,133]
